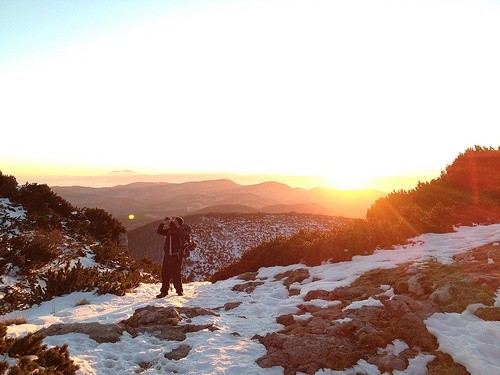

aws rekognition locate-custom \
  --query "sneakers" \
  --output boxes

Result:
[156,292,168,298]
[179,291,183,295]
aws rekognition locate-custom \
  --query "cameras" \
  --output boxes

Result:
[166,217,170,220]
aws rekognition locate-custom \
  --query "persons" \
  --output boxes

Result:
[156,216,184,299]
